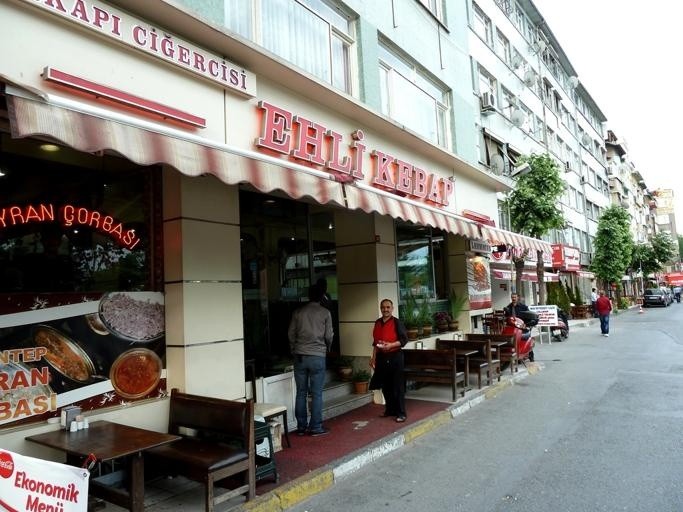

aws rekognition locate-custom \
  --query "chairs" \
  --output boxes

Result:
[479,308,507,335]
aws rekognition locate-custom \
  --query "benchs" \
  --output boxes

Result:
[375,332,521,405]
[138,387,262,512]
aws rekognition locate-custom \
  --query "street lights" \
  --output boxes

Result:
[504,161,532,295]
[638,241,650,293]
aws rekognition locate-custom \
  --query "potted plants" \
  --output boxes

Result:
[564,277,591,320]
[447,288,469,333]
[349,368,373,394]
[337,354,354,382]
[400,281,422,341]
[418,292,433,339]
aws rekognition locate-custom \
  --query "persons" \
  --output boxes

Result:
[368,299,407,421]
[596,290,610,336]
[591,288,596,317]
[673,286,680,303]
[505,294,534,362]
[318,279,332,307]
[288,285,334,437]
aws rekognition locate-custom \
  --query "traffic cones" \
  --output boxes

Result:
[637,303,643,313]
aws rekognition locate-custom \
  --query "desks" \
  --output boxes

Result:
[17,414,184,512]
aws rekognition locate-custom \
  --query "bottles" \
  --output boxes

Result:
[483,324,485,334]
[70,415,90,433]
[486,325,490,335]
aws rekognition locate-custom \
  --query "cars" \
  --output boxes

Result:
[642,287,673,307]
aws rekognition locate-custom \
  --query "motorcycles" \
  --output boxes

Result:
[551,306,569,341]
[498,306,540,372]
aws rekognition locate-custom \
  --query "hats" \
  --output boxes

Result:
[307,278,327,302]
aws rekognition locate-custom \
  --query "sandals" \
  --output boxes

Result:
[379,409,406,423]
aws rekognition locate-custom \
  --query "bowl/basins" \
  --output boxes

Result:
[376,344,385,349]
[47,416,60,426]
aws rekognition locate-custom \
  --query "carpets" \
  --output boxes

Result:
[221,394,458,499]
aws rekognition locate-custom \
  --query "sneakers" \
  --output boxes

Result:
[297,426,330,436]
